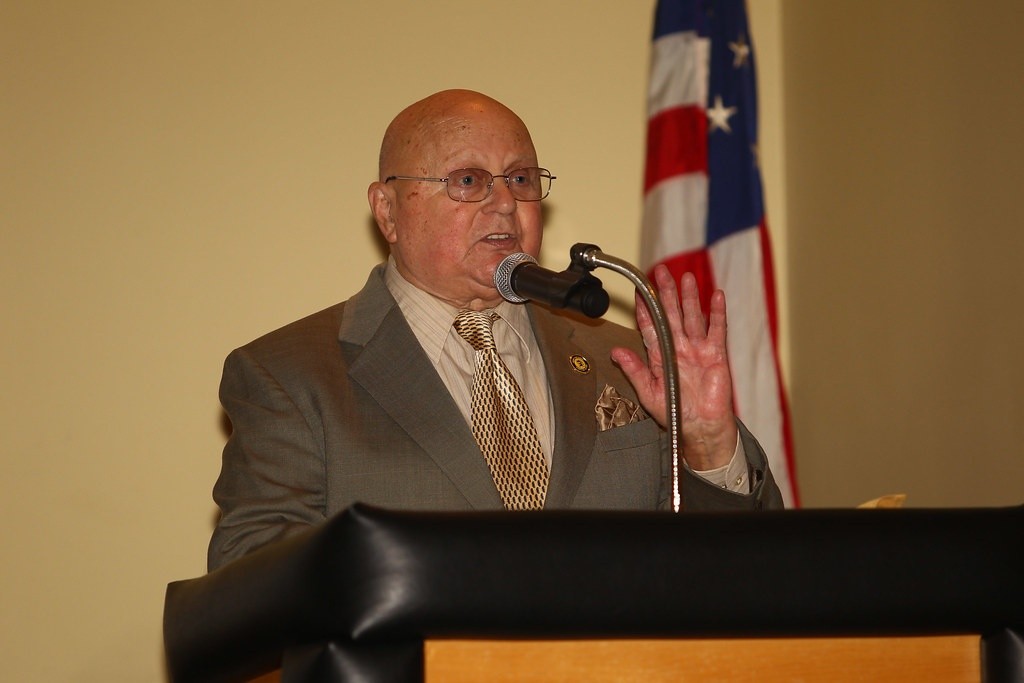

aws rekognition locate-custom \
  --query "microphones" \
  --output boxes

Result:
[494,252,610,319]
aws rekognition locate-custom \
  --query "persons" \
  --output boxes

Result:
[208,89,783,571]
[639,1,802,507]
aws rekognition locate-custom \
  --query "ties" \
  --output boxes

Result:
[452,310,549,509]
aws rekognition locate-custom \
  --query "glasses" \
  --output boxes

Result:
[384,166,557,203]
